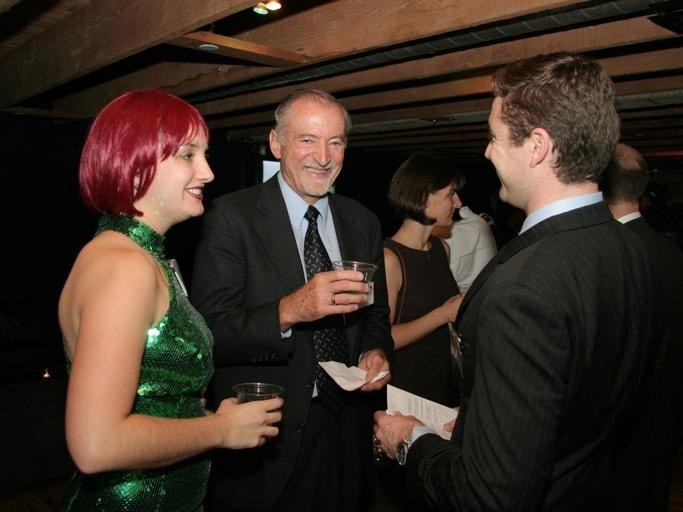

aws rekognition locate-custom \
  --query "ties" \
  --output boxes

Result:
[304,205,350,415]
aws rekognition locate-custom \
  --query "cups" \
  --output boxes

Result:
[232,384,285,405]
[332,260,377,296]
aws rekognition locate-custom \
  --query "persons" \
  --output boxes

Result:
[58,52,682,510]
